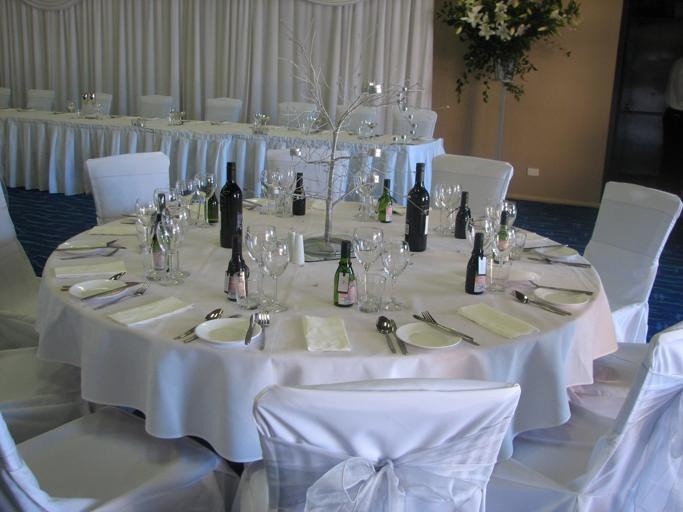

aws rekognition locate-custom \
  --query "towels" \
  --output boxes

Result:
[87,225,135,237]
[54,259,127,279]
[457,303,538,341]
[106,295,192,328]
[301,312,352,352]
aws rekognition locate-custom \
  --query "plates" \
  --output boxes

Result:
[55,169,591,358]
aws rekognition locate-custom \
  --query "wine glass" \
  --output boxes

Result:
[2,98,399,141]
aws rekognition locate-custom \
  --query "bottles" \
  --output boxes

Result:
[217,160,243,249]
[404,163,430,253]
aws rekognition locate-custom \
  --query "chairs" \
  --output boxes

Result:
[430,150,516,215]
[27,89,56,113]
[234,380,525,512]
[277,103,437,140]
[204,97,244,123]
[1,344,93,442]
[0,405,240,512]
[488,318,683,512]
[0,87,12,107]
[81,93,113,115]
[85,151,170,225]
[135,94,175,120]
[581,182,683,346]
[0,182,48,348]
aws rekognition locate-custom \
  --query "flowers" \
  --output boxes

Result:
[438,0,584,104]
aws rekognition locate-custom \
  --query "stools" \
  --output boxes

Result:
[587,340,650,386]
[530,377,635,443]
